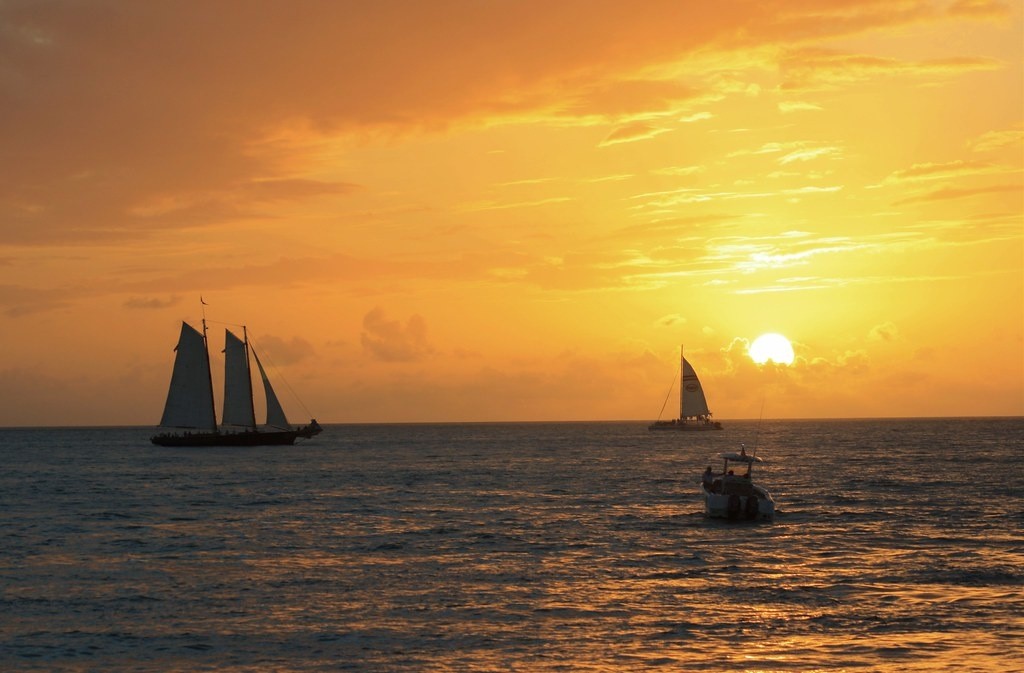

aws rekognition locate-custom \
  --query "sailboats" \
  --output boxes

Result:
[647,343,725,433]
[150,292,326,448]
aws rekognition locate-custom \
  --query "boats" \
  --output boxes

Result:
[698,401,777,523]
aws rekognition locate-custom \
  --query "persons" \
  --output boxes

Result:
[702,466,725,493]
[727,470,733,476]
